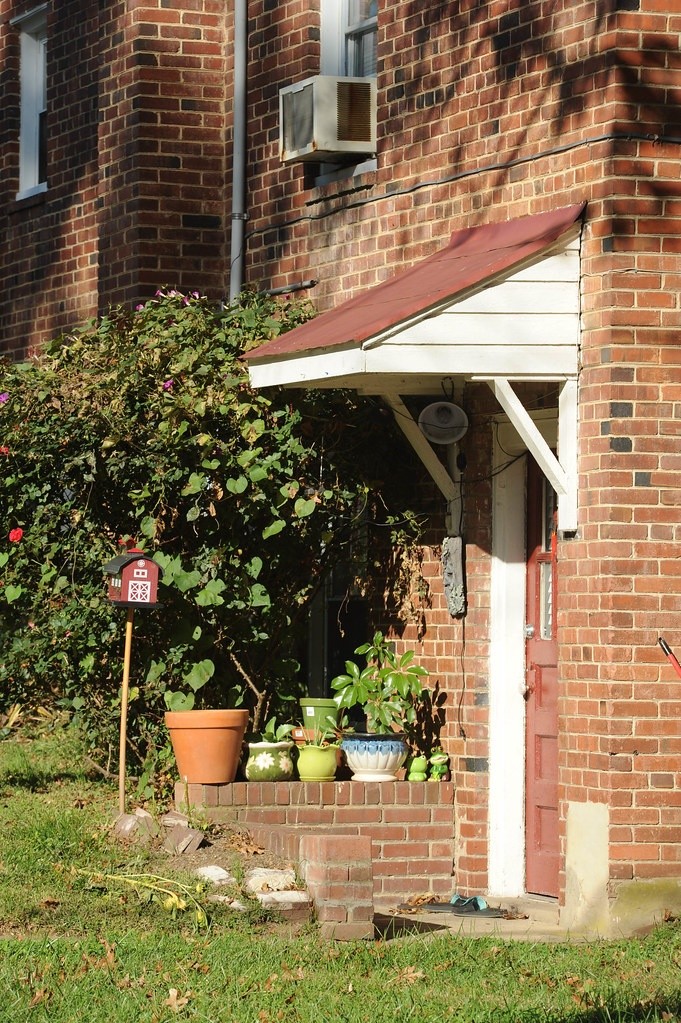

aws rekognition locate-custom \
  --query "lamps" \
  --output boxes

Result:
[417,402,469,446]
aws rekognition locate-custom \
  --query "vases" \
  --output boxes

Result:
[164,708,250,786]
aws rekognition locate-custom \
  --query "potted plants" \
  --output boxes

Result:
[289,712,340,782]
[330,630,434,784]
[239,715,297,783]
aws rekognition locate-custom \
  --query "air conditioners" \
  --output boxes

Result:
[278,74,379,167]
